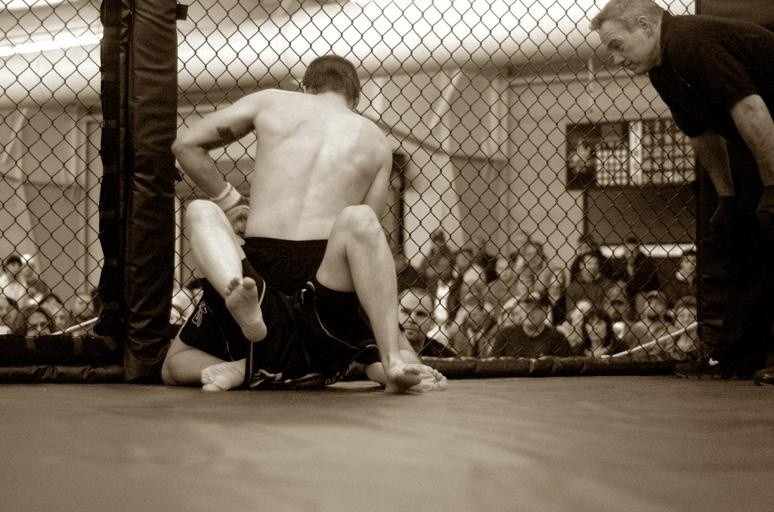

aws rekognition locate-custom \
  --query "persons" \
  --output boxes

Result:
[587,0,774,385]
[160,55,449,391]
[384,228,696,355]
[0,252,98,338]
[167,274,206,325]
[181,199,423,394]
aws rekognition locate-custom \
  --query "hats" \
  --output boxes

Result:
[646,290,668,303]
[521,288,554,307]
[605,286,626,305]
[674,293,699,308]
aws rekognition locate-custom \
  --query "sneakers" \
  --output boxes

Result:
[672,349,774,386]
